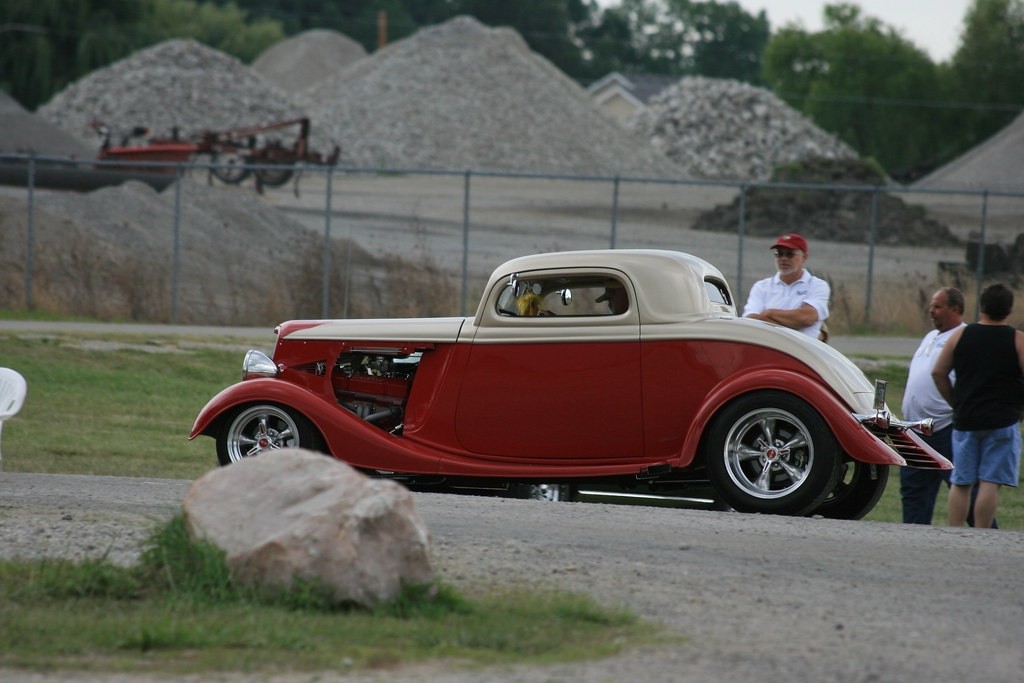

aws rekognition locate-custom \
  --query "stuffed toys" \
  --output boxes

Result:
[517,293,541,317]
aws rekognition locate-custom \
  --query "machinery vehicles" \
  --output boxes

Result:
[91,116,339,192]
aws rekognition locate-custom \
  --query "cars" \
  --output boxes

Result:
[187,249,956,521]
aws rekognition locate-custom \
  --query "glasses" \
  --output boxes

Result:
[773,250,804,258]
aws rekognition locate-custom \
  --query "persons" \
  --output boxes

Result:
[602,282,630,314]
[742,233,831,342]
[898,283,1024,529]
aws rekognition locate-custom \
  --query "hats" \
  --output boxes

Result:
[595,280,625,302]
[771,234,808,254]
